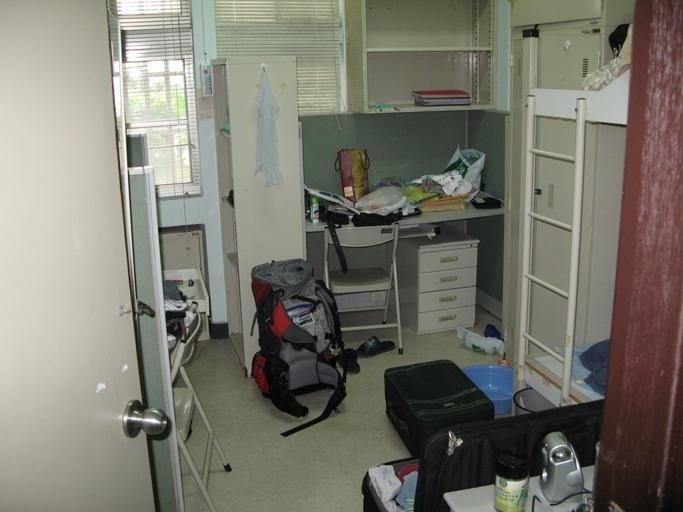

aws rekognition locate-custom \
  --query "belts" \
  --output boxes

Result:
[327,217,347,274]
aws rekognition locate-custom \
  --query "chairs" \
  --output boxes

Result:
[172,301,232,512]
[322,222,405,356]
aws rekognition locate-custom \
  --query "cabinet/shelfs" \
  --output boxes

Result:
[210,55,308,379]
[305,0,510,232]
[400,238,481,334]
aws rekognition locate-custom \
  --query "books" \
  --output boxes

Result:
[417,196,469,210]
[413,97,471,105]
[410,89,469,99]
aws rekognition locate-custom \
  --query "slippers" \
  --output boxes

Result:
[354,335,395,359]
[337,348,359,373]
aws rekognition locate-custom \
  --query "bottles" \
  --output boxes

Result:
[310,195,320,224]
[495,452,531,512]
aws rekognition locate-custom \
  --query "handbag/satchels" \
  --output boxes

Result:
[334,148,370,202]
[352,207,422,226]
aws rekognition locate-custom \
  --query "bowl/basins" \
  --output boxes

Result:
[461,364,515,415]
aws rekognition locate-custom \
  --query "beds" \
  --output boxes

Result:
[513,32,630,417]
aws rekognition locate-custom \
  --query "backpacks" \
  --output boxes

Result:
[250,258,346,437]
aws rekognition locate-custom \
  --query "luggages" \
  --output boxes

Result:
[361,397,607,512]
[383,360,494,457]
